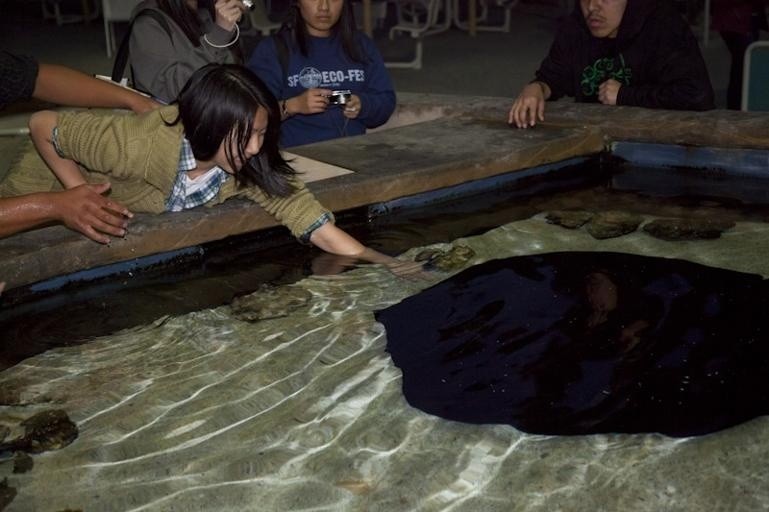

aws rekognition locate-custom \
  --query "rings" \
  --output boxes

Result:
[352,106,357,112]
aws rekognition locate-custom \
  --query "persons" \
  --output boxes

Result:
[128,0,247,108]
[0,46,169,245]
[506,0,717,131]
[0,63,442,285]
[238,0,396,154]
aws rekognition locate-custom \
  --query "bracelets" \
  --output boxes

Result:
[534,79,546,95]
[282,97,292,119]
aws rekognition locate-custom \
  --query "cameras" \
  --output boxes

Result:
[236,1,254,11]
[323,88,351,109]
